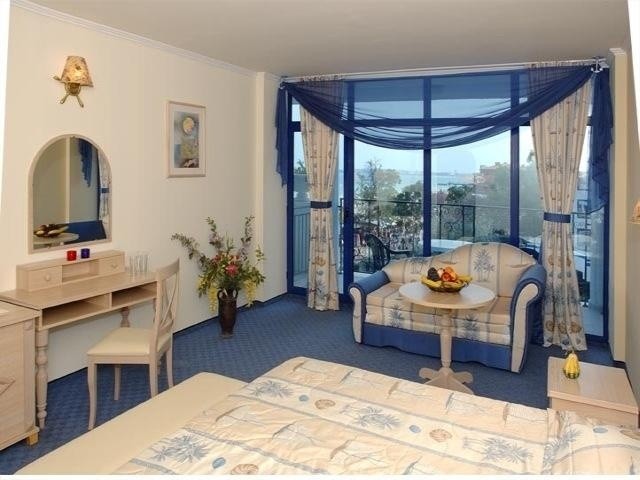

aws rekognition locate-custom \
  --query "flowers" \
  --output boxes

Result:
[167,211,267,316]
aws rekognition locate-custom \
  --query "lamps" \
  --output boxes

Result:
[53,55,94,107]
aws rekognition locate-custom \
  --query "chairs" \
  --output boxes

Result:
[84,256,179,430]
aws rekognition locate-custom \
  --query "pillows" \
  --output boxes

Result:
[541,403,640,478]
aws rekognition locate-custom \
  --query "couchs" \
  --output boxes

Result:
[342,239,546,375]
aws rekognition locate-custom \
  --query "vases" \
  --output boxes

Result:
[218,290,238,337]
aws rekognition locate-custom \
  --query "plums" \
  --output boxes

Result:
[442,273,452,282]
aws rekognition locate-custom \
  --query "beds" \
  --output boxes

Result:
[12,369,639,477]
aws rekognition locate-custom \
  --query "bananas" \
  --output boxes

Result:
[421,277,441,288]
[458,275,473,282]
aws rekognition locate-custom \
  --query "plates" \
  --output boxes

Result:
[34,230,60,238]
[424,277,466,293]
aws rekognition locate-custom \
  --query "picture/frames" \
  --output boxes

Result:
[166,97,207,179]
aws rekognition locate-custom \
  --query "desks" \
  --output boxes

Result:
[0,268,160,449]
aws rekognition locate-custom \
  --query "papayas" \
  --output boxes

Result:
[562,346,581,379]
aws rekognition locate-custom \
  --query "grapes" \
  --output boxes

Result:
[427,267,440,282]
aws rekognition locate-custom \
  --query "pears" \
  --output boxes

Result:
[444,266,457,281]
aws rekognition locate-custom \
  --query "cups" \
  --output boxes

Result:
[127,256,140,283]
[138,254,150,280]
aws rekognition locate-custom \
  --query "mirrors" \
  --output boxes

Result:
[27,134,114,255]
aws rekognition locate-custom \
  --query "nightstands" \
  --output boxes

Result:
[545,355,640,421]
